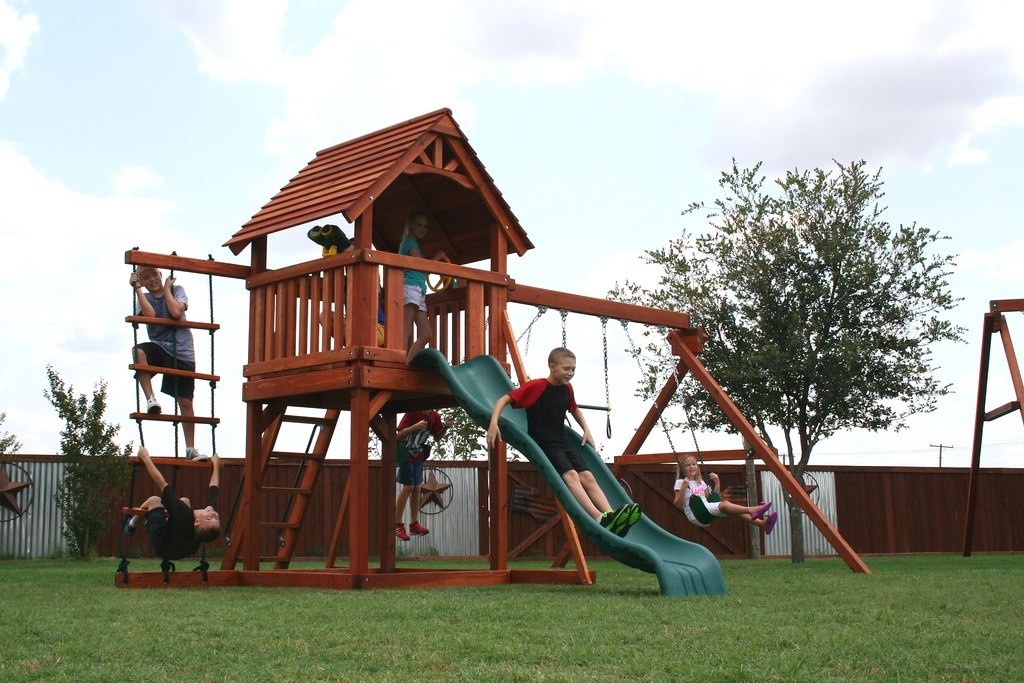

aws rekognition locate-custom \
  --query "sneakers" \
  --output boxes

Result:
[185,448,209,462]
[395,523,410,541]
[124,516,135,536]
[146,397,162,414]
[408,521,429,537]
[597,504,631,532]
[614,501,641,538]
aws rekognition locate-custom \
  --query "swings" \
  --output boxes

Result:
[620,320,723,526]
[559,310,612,413]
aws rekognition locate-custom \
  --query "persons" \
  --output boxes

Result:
[395,411,454,541]
[486,346,643,539]
[673,453,777,535]
[123,445,221,561]
[130,265,210,463]
[318,237,386,348]
[398,209,445,366]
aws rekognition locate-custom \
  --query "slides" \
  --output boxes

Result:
[405,346,727,596]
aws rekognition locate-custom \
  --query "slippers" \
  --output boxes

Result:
[751,501,773,520]
[761,512,777,535]
